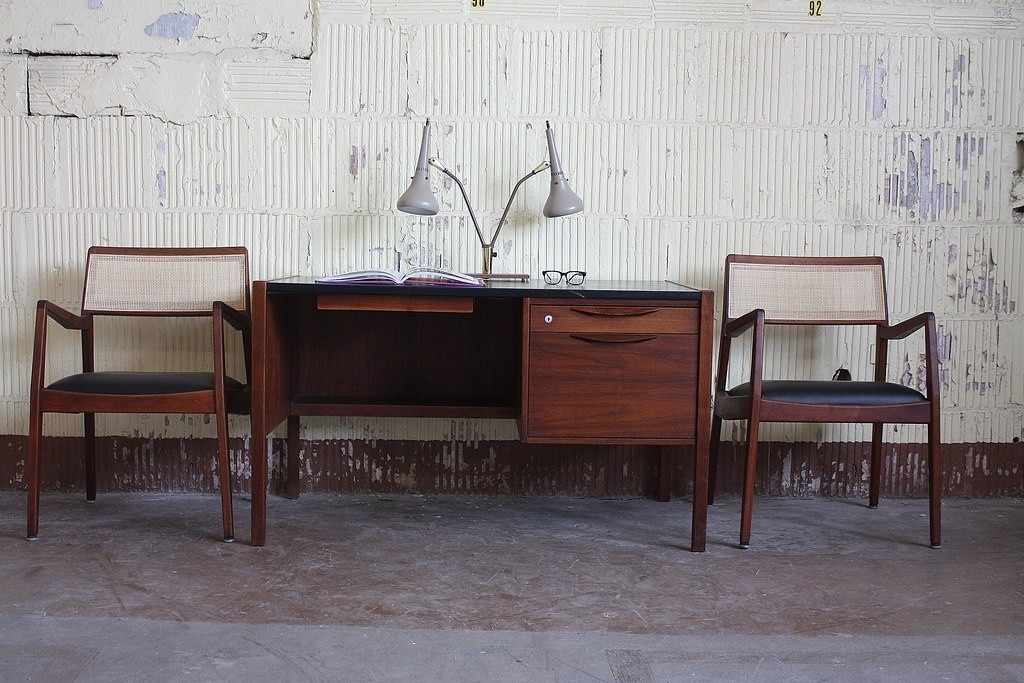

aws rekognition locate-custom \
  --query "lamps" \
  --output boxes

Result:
[395,117,584,281]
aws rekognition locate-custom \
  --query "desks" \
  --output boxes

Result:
[250,274,715,553]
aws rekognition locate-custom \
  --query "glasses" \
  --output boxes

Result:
[542,271,587,286]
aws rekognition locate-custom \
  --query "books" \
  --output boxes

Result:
[315,266,487,287]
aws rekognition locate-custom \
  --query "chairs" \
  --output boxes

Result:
[28,247,253,542]
[707,253,942,550]
